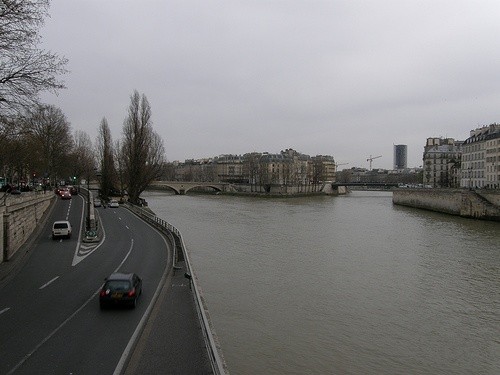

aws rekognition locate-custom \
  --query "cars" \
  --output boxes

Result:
[96,171,103,176]
[57,187,73,200]
[99,273,144,310]
[52,221,73,240]
[94,198,102,207]
[70,188,77,194]
[107,200,119,208]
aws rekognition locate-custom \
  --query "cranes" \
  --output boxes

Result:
[366,154,382,171]
[335,161,350,172]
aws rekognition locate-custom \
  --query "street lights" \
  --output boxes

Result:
[87,168,98,230]
[32,171,36,187]
[73,176,76,185]
